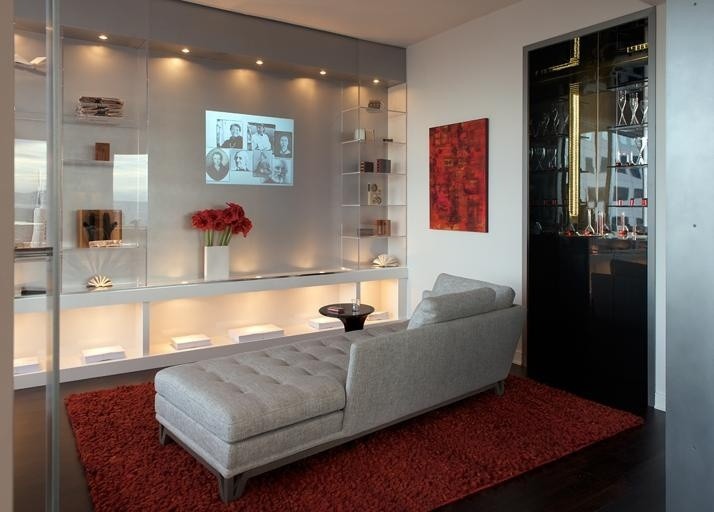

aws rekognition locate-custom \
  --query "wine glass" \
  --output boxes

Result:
[618,88,648,126]
[634,137,646,166]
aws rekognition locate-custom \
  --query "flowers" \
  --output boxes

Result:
[189,200,253,248]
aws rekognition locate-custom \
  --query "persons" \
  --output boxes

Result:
[249,123,271,152]
[221,123,242,148]
[264,160,288,184]
[276,134,290,155]
[232,150,249,171]
[253,153,271,176]
[207,152,227,180]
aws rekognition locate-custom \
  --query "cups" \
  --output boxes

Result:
[353,128,365,139]
[364,129,374,141]
[616,149,634,167]
[351,299,361,312]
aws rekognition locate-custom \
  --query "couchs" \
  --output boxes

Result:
[145,264,526,507]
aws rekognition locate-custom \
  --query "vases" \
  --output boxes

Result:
[202,243,231,283]
[29,206,48,250]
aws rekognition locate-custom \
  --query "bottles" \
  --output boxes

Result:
[376,219,383,236]
[564,213,576,236]
[383,220,391,238]
[616,212,629,236]
[641,198,648,206]
[630,198,635,206]
[585,209,595,235]
[617,199,625,207]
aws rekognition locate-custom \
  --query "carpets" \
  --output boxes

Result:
[59,365,650,512]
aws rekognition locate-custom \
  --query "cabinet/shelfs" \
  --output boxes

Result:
[525,82,592,206]
[605,71,650,215]
[58,109,143,253]
[334,72,408,274]
[522,221,657,418]
[6,59,53,304]
